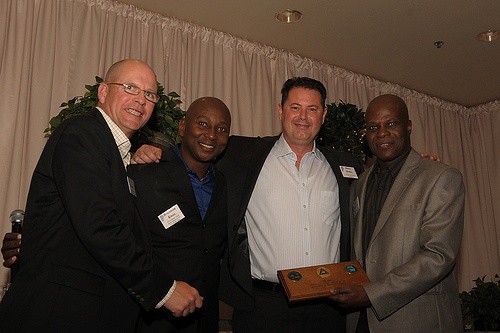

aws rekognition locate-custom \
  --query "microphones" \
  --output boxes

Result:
[9,209,25,282]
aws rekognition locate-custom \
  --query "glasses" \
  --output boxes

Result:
[107,81,160,105]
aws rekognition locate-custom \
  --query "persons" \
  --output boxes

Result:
[129,77,442,333]
[330,94,465,333]
[1,97,249,333]
[0,58,204,333]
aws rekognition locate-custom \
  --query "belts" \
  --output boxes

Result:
[252,278,282,293]
